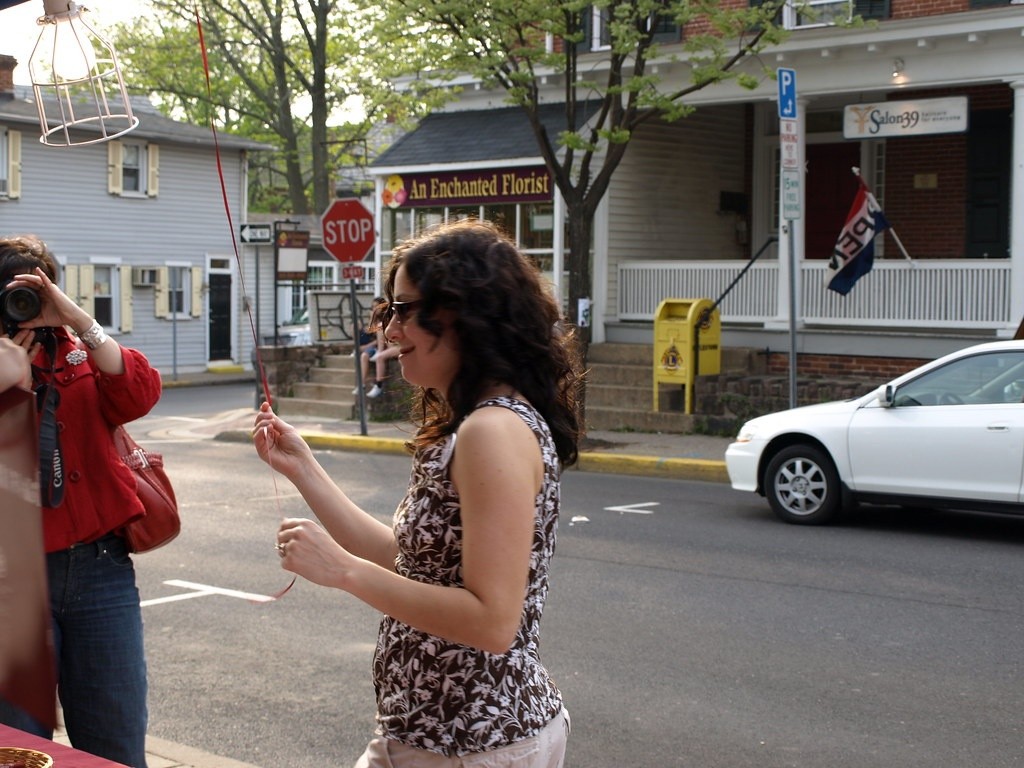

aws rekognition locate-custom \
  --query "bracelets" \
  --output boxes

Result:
[77,319,108,350]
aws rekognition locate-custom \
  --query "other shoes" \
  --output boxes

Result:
[352,384,366,396]
[366,384,383,398]
[369,349,382,362]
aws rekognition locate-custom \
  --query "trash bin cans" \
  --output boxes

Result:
[652,299,721,414]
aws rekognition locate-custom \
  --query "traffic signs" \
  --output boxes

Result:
[239,223,273,246]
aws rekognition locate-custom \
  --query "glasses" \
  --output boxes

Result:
[389,299,428,324]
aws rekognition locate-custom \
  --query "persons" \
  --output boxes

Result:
[250,218,588,768]
[349,298,401,398]
[0,233,162,768]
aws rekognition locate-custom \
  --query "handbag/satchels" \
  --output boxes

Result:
[73,336,180,553]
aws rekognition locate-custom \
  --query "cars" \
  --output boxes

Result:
[259,307,314,347]
[724,340,1024,526]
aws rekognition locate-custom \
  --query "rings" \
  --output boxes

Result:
[40,284,45,289]
[276,542,287,558]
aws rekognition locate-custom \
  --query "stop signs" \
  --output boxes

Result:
[320,197,375,265]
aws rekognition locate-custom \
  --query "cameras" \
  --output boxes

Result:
[0,286,43,325]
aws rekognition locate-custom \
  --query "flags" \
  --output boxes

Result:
[823,167,893,297]
